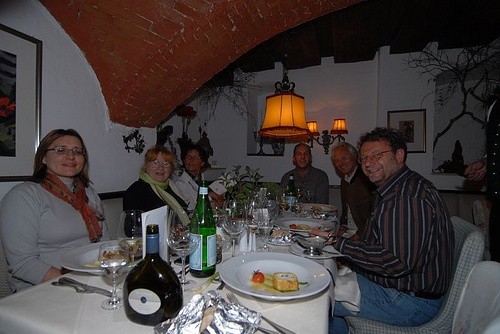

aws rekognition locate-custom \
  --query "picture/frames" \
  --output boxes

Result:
[385,109,427,154]
[0,24,43,183]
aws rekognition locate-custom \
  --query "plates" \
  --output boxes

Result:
[278,218,334,236]
[218,253,331,301]
[266,236,293,246]
[218,252,331,302]
[303,204,337,212]
[60,239,120,277]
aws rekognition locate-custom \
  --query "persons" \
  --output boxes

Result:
[463,99,500,262]
[281,143,329,204]
[331,142,375,240]
[309,127,455,334]
[0,129,112,292]
[174,142,225,209]
[123,147,190,235]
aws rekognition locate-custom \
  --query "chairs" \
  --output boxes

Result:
[472,198,492,259]
[450,261,500,334]
[344,216,486,334]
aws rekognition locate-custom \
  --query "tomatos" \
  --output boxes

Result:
[252,270,264,283]
[289,224,297,229]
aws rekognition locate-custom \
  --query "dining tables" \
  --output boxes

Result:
[0,201,337,334]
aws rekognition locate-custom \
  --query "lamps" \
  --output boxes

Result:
[308,118,349,155]
[258,32,313,143]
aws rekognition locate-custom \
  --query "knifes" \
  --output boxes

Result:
[60,277,112,297]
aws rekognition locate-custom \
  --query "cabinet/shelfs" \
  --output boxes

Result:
[223,183,493,260]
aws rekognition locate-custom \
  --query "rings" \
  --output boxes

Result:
[327,233,332,239]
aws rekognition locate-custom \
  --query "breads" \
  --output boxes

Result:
[298,224,310,230]
[273,272,299,291]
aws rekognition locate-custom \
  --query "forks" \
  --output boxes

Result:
[226,291,296,334]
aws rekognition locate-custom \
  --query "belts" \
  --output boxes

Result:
[403,290,441,300]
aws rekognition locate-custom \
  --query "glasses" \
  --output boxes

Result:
[357,149,393,165]
[184,154,201,160]
[47,145,86,157]
[148,160,172,168]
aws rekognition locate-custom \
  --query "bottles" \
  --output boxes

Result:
[190,180,217,278]
[283,175,298,214]
[124,224,184,327]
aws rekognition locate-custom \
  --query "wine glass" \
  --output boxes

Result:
[245,199,263,253]
[118,210,145,268]
[221,200,247,259]
[261,200,277,253]
[100,242,129,309]
[166,210,197,292]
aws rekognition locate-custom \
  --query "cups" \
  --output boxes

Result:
[247,190,310,225]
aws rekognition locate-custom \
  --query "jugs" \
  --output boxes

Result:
[291,233,329,257]
